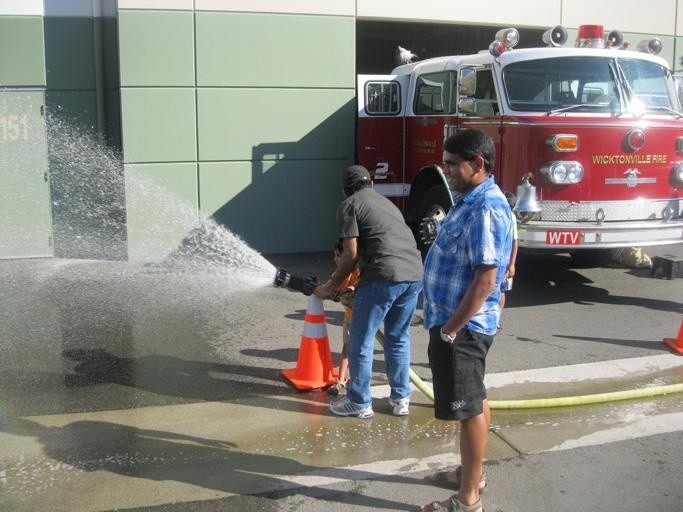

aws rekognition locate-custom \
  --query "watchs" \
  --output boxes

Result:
[439,328,457,344]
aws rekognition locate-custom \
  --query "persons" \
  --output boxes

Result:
[315,163,425,421]
[493,190,518,331]
[418,129,514,512]
[313,236,360,400]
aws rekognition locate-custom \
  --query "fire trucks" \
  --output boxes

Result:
[356,25,683,264]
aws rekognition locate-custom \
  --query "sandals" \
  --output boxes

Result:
[418,495,485,512]
[327,379,348,396]
[424,466,488,493]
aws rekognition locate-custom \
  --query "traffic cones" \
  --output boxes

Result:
[664,320,683,354]
[281,294,339,390]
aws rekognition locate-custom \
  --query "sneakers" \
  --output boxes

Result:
[387,397,410,416]
[330,399,375,419]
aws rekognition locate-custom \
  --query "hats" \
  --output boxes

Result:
[341,164,370,187]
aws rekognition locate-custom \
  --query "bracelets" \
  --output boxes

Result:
[330,276,340,287]
